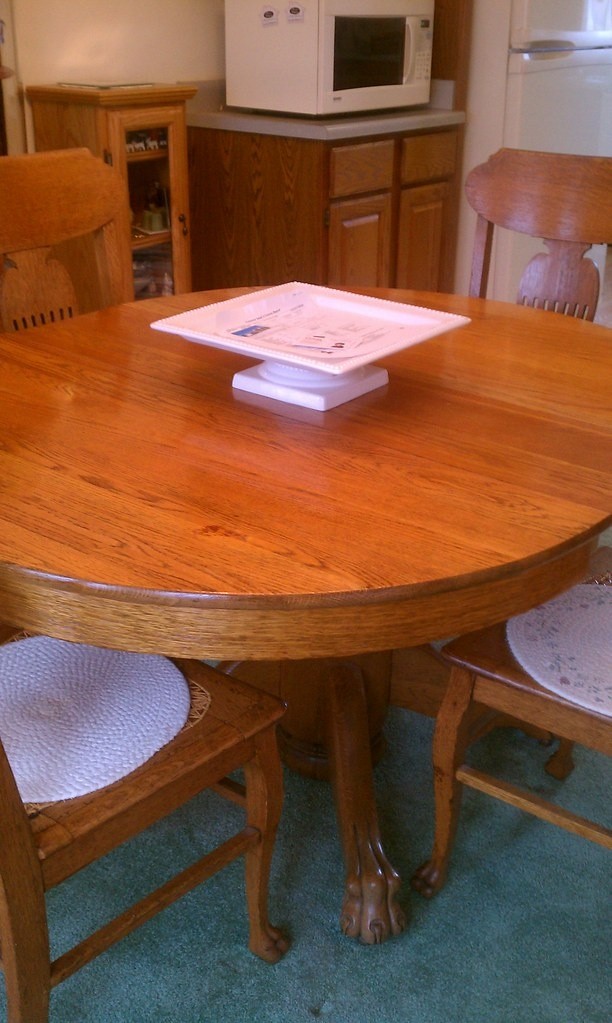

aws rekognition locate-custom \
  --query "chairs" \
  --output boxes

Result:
[463,148,612,329]
[0,148,129,336]
[0,620,294,1023]
[409,542,612,900]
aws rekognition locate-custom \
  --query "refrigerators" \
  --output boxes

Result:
[454,0,612,323]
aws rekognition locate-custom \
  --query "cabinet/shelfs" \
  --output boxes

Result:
[185,109,467,310]
[24,80,198,304]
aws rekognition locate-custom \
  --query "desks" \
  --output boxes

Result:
[0,284,612,945]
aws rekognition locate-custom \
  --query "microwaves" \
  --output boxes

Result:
[225,0,432,117]
